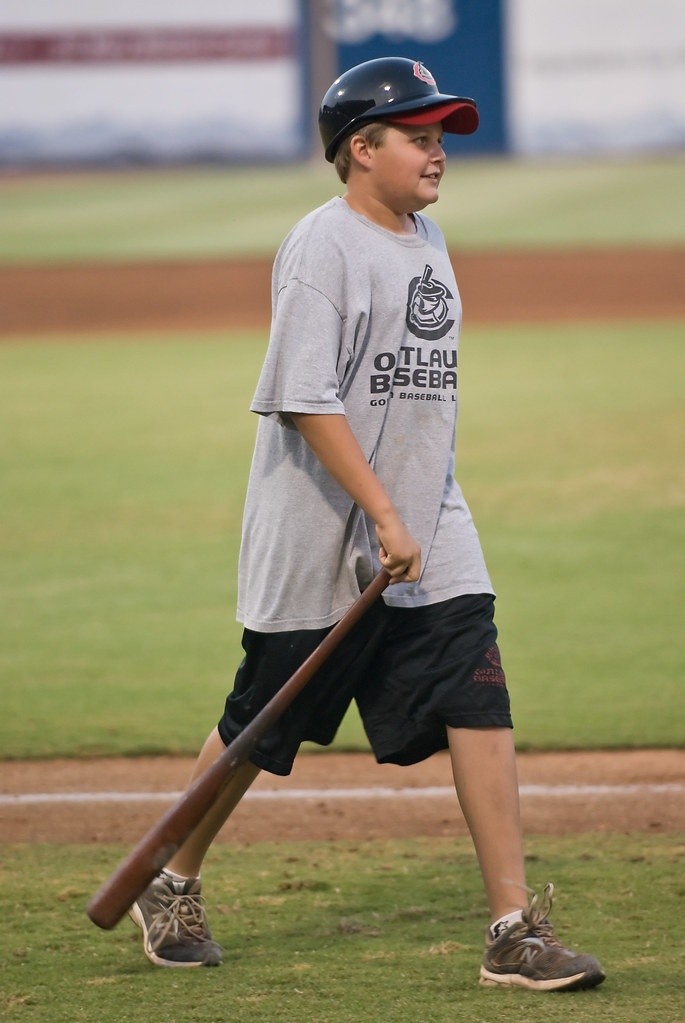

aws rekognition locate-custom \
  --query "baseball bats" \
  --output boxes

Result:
[86,566,393,931]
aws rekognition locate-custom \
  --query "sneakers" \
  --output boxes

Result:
[481,879,606,992]
[127,871,226,967]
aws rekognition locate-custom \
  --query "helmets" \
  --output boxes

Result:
[318,57,478,163]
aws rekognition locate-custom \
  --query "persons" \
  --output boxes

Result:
[128,57,605,989]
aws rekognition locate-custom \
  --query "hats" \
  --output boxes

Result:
[382,100,479,135]
